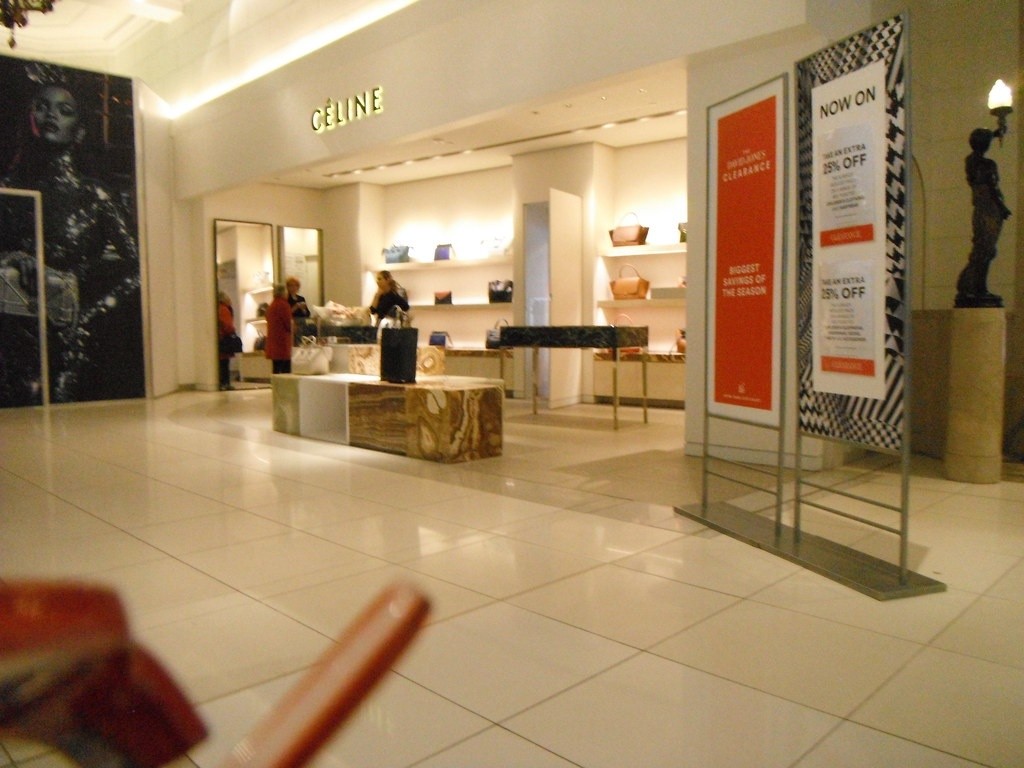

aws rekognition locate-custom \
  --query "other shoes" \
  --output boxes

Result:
[220,385,235,390]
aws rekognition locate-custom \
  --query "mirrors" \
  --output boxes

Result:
[277,226,325,315]
[213,217,276,391]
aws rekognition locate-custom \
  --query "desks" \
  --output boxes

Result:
[500,324,650,430]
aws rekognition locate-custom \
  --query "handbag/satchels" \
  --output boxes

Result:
[489,281,512,302]
[608,315,639,352]
[290,335,334,375]
[1,267,78,325]
[395,283,408,304]
[670,328,686,354]
[486,319,511,349]
[428,331,455,347]
[609,213,649,246]
[434,244,454,260]
[385,245,410,262]
[680,271,686,288]
[610,264,650,299]
[220,333,243,353]
[677,222,686,242]
[435,291,451,305]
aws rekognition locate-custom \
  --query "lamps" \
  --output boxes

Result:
[987,79,1014,148]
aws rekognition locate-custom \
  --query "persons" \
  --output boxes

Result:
[284,277,310,347]
[370,271,410,343]
[955,128,1013,309]
[0,64,140,404]
[265,283,293,373]
[218,292,236,390]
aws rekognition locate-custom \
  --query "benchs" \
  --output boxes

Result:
[237,351,273,382]
[272,372,504,461]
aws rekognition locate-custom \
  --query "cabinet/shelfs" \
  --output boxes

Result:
[372,254,514,355]
[593,242,689,363]
